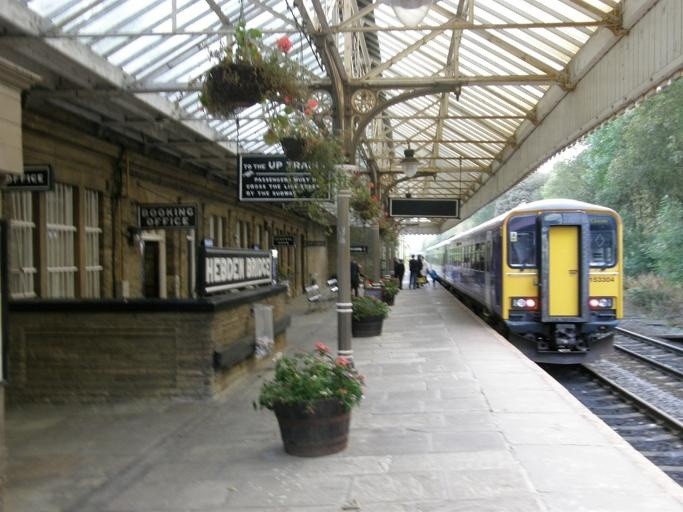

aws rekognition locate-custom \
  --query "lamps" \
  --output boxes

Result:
[399,142,421,177]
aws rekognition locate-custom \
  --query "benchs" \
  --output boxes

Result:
[305,278,339,313]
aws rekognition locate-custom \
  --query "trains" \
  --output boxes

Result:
[414,198,623,364]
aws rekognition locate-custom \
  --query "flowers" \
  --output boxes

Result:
[192,15,389,226]
[351,295,392,321]
[252,340,368,417]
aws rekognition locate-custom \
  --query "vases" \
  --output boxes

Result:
[352,315,385,336]
[272,397,352,458]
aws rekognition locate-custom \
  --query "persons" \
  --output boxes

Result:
[350,255,364,297]
[395,258,404,289]
[415,253,426,288]
[392,256,398,277]
[407,253,418,290]
